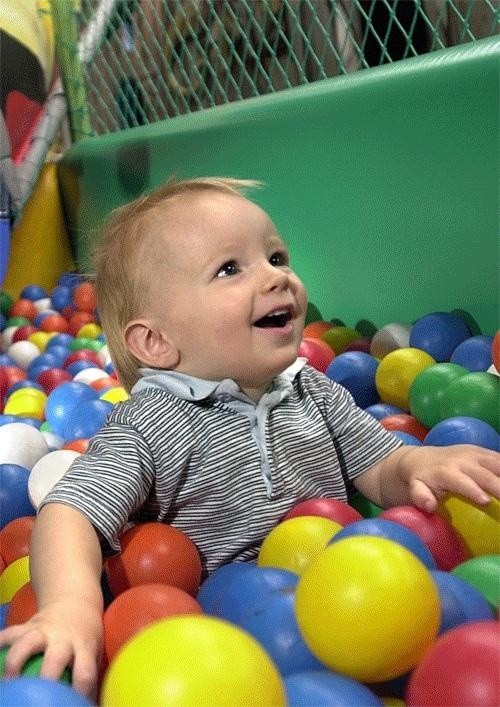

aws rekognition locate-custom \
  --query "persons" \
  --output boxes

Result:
[1,3,57,278]
[1,174,500,696]
